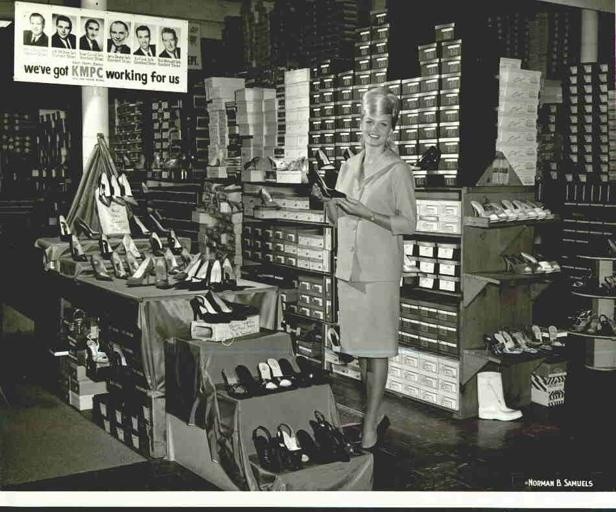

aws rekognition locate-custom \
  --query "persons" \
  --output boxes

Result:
[159,27,180,59]
[80,19,101,51]
[52,15,76,48]
[23,13,48,47]
[133,25,155,56]
[308,87,416,454]
[107,20,131,54]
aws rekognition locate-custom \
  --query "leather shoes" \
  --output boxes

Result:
[571,272,616,297]
[343,148,354,160]
[258,188,278,208]
[62,305,127,367]
[252,409,351,473]
[309,163,347,199]
[315,149,336,170]
[567,307,615,336]
[482,322,566,360]
[242,152,306,171]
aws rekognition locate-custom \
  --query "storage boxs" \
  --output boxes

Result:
[201,0,616,422]
[108,89,207,255]
[49,296,154,459]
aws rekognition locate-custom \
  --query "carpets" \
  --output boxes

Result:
[1,374,149,487]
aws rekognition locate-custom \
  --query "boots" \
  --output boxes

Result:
[477,371,525,422]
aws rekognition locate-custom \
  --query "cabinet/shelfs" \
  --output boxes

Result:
[31,236,377,492]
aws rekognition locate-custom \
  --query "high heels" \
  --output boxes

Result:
[407,145,441,172]
[503,247,561,275]
[190,288,260,324]
[54,147,245,292]
[470,195,555,223]
[348,414,392,455]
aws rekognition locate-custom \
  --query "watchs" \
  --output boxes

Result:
[370,212,376,222]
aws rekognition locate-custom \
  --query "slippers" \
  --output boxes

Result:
[221,354,328,400]
